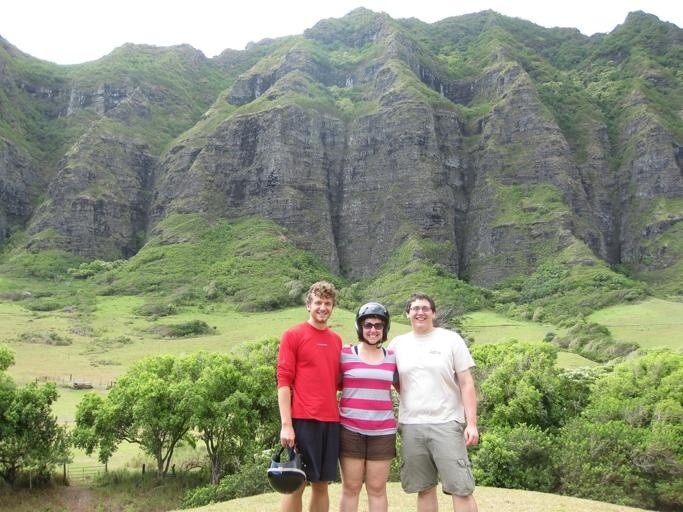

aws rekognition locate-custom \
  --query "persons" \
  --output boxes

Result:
[386,292,480,512]
[275,281,342,511]
[290,301,400,511]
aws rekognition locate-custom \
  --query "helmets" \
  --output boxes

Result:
[354,302,391,342]
[268,452,307,495]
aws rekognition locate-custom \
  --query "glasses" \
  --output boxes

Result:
[360,322,385,329]
